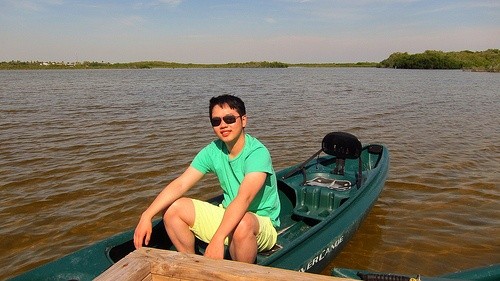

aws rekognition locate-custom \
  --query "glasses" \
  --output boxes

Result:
[208,113,246,127]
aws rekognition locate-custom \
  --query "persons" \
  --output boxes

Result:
[133,94,281,263]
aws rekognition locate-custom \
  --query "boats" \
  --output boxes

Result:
[331,262,500,281]
[0,131,390,281]
